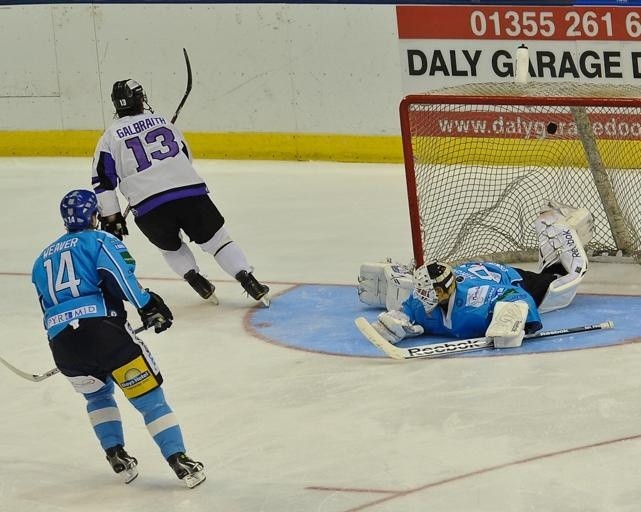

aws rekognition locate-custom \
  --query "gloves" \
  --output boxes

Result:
[137,292,173,333]
[98,213,128,241]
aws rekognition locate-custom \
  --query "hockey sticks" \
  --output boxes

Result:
[1,316,163,382]
[354,316,614,361]
[121,47,193,221]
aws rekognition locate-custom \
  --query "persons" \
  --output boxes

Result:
[31,189,204,479]
[356,197,596,351]
[91,80,271,300]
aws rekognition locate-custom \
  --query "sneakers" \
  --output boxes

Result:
[235,270,269,300]
[106,447,138,473]
[168,453,204,479]
[183,269,215,299]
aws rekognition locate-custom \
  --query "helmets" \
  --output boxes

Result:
[111,78,147,117]
[60,190,98,230]
[415,259,456,296]
